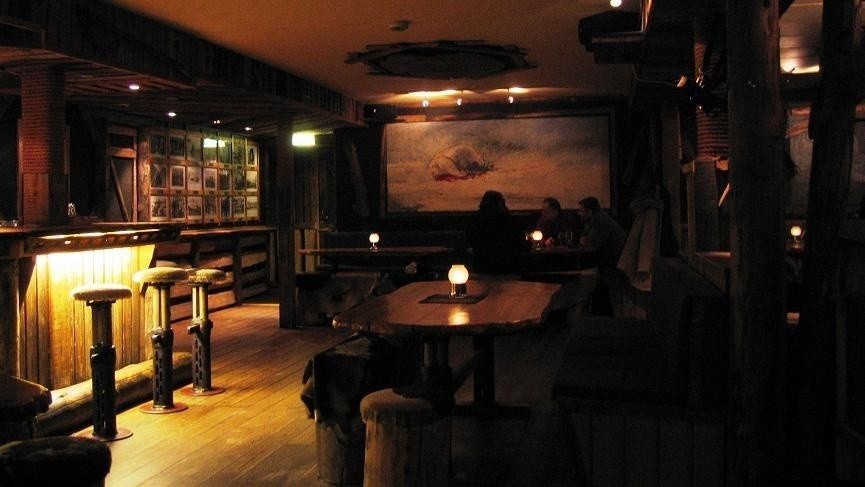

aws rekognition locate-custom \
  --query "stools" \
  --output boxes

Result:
[70,264,229,441]
[311,377,459,487]
[287,271,418,328]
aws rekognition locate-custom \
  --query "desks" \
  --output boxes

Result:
[333,282,563,487]
[297,243,454,288]
[513,244,599,268]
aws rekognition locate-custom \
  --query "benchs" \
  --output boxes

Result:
[316,230,470,274]
[555,256,730,486]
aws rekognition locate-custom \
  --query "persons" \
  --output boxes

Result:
[464,191,532,273]
[534,197,581,243]
[578,197,628,268]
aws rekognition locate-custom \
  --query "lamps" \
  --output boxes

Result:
[446,261,471,299]
